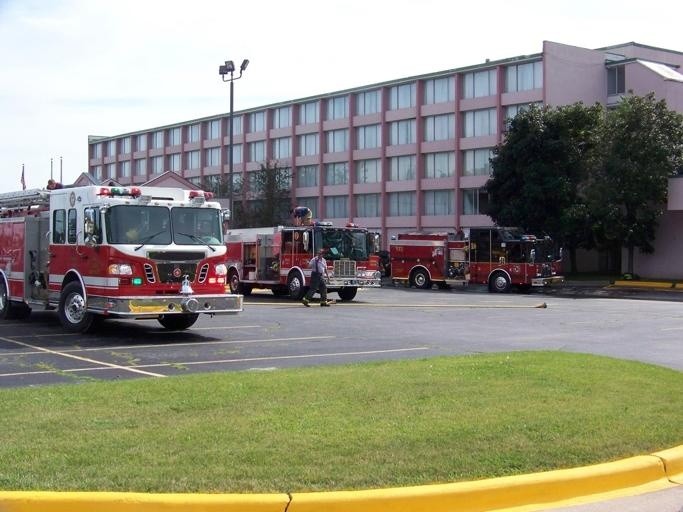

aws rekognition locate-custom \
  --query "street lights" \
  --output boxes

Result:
[218,59,250,223]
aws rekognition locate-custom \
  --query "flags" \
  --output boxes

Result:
[21,166,26,189]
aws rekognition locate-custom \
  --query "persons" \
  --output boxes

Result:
[47,179,63,190]
[302,249,330,307]
[289,206,313,225]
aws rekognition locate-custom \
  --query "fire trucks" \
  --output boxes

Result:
[389,223,565,293]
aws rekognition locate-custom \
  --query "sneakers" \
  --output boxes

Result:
[320,303,330,306]
[302,300,309,307]
